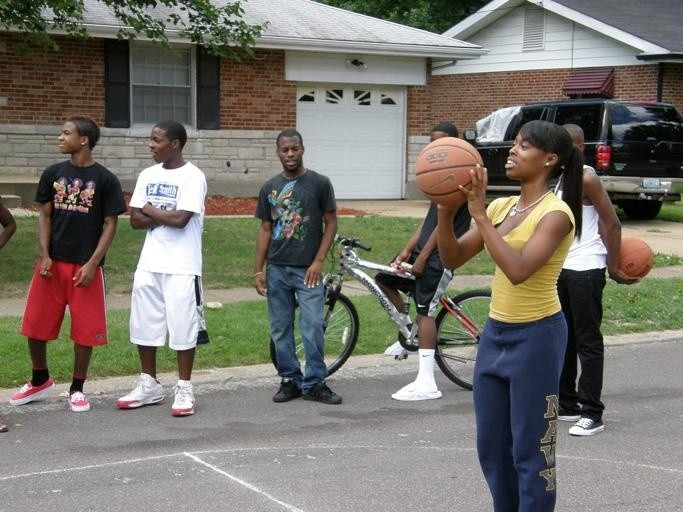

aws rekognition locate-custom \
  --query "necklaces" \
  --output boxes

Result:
[505,187,556,216]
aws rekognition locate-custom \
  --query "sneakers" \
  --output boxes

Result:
[384,341,419,355]
[302,383,342,404]
[274,378,302,402]
[392,382,442,401]
[115,372,164,408]
[69,392,89,412]
[556,408,582,422]
[10,377,55,407]
[171,381,195,416]
[569,418,604,435]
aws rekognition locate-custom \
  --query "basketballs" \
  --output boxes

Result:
[415,137,485,208]
[619,237,653,279]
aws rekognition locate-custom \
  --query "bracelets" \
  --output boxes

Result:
[253,270,264,278]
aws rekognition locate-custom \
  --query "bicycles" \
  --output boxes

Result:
[269,234,492,392]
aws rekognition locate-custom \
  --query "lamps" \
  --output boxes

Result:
[348,60,368,70]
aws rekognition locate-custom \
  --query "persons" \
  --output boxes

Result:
[251,127,349,407]
[371,121,469,407]
[113,119,212,419]
[5,115,131,425]
[432,119,577,511]
[552,123,639,436]
[0,200,17,432]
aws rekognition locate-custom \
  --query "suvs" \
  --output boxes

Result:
[461,99,682,219]
